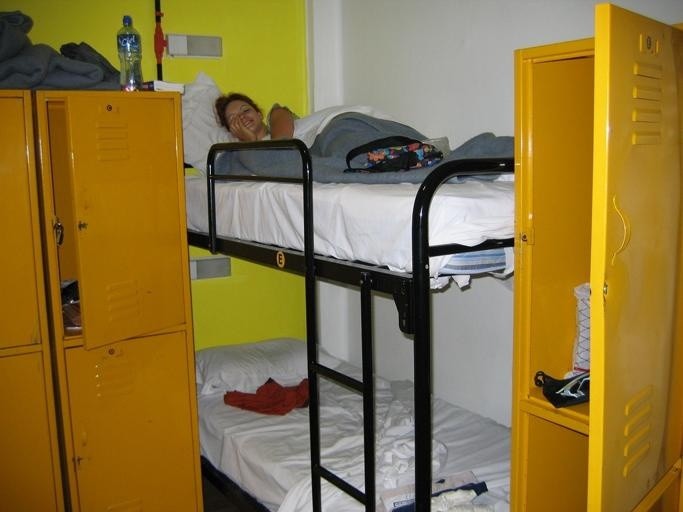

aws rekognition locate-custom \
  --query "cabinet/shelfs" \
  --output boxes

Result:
[36,88,206,512]
[509,3,682,512]
[0,88,66,511]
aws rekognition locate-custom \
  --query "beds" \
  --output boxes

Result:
[185,141,514,512]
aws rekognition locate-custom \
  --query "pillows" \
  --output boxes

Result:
[195,338,341,399]
[179,71,239,175]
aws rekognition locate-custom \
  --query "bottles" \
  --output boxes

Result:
[116,15,144,92]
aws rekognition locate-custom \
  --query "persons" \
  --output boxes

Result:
[212,92,300,142]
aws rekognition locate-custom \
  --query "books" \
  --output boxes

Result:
[381,469,488,512]
[121,80,184,95]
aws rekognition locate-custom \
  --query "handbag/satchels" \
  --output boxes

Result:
[367,143,443,169]
[535,371,589,409]
